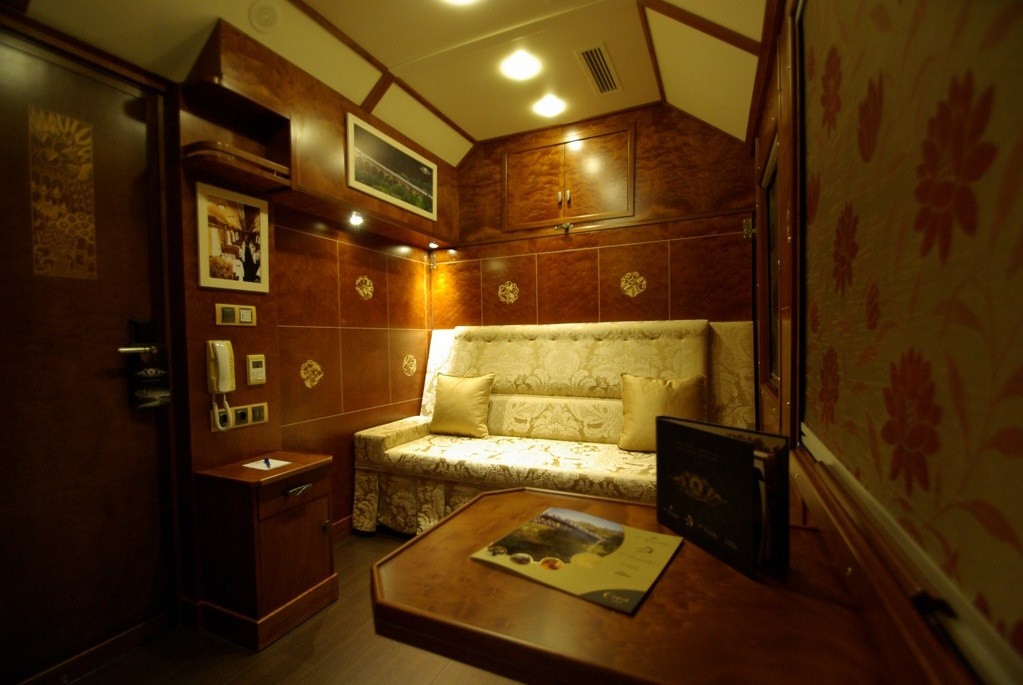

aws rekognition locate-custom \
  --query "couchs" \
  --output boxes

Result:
[353,317,757,534]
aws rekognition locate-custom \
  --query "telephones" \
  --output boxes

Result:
[206,339,237,394]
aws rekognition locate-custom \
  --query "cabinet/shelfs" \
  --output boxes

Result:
[180,449,340,648]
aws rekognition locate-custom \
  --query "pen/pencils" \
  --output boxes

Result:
[264,458,270,468]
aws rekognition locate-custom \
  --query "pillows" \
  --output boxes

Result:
[618,372,705,453]
[428,371,494,439]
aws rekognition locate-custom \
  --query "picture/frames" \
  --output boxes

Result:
[345,111,439,223]
[197,181,270,293]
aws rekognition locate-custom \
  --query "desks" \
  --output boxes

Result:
[370,485,932,685]
